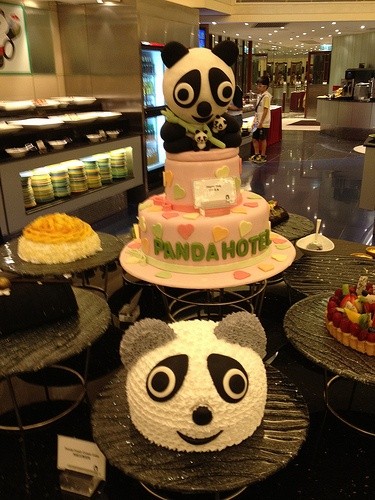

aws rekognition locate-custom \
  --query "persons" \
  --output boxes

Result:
[248,76,272,163]
[225,84,244,133]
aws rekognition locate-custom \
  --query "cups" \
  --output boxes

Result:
[366,247,375,259]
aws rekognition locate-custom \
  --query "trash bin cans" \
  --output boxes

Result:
[268,104,282,145]
[290,91,305,112]
[359,134,375,211]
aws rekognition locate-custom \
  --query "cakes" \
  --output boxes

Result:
[17,211,101,264]
[119,312,267,453]
[326,275,375,357]
[127,40,271,275]
[267,199,289,228]
[0,272,80,338]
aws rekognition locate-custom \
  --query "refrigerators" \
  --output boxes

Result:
[140,40,168,191]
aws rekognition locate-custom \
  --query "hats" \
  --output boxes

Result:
[255,75,269,89]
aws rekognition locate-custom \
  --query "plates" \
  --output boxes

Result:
[19,150,128,208]
[0,96,121,133]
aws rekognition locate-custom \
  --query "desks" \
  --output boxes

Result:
[284,292,375,458]
[248,211,315,314]
[283,237,375,305]
[0,282,113,499]
[0,233,124,302]
[92,360,311,500]
[122,271,268,322]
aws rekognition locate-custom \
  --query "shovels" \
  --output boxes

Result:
[307,219,323,250]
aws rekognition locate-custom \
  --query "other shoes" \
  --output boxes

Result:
[249,154,267,163]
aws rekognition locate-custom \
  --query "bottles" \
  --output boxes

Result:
[145,123,157,165]
[142,56,155,94]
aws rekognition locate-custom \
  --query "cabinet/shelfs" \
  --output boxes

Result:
[0,103,143,239]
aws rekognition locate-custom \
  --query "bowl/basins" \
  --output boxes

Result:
[86,134,102,143]
[106,131,119,139]
[5,147,28,159]
[48,140,67,149]
[295,233,335,257]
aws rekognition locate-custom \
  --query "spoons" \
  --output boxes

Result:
[306,219,323,250]
